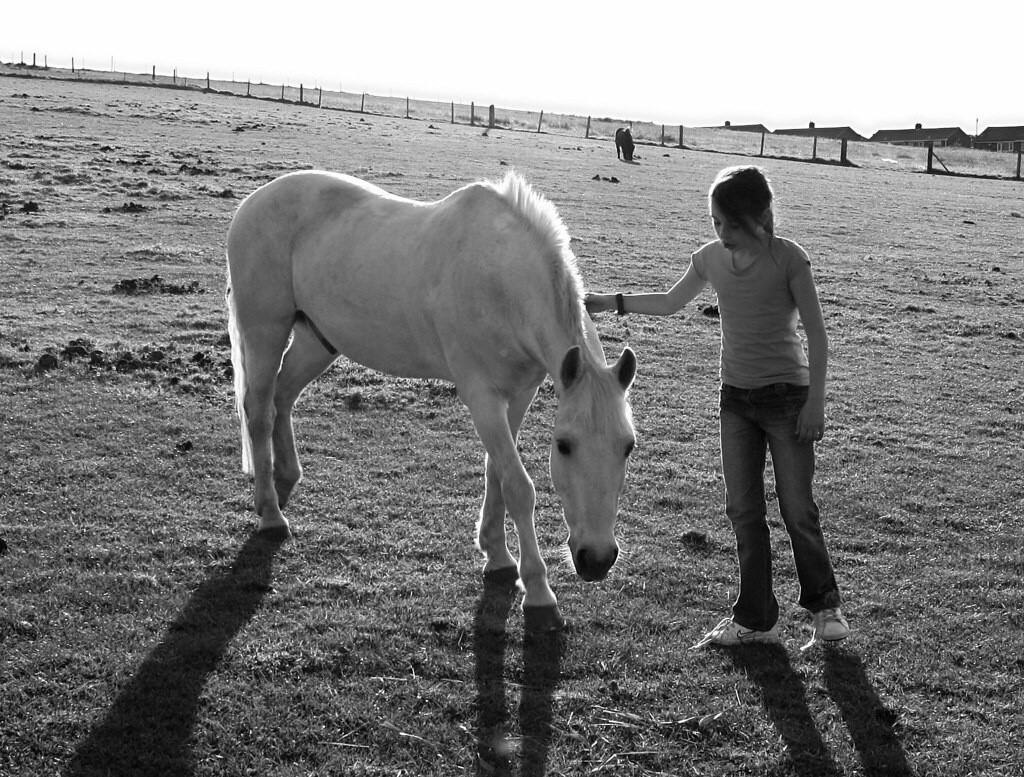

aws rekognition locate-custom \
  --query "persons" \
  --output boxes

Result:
[582,163,851,649]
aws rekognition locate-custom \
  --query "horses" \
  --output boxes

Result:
[226,168,635,633]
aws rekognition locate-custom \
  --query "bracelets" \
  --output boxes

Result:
[616,293,627,316]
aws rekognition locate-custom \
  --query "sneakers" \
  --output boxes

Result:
[799,607,850,653]
[688,617,779,650]
